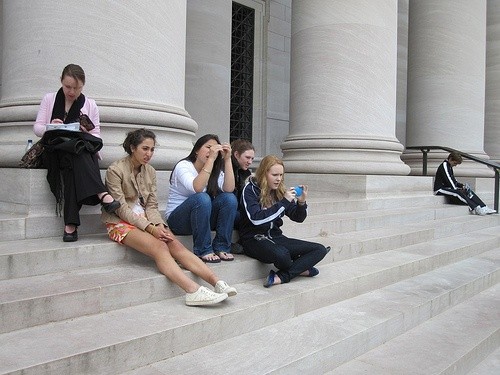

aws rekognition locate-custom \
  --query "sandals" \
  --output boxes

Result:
[199,253,221,263]
[218,252,234,260]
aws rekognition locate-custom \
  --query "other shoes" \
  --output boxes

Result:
[64,226,78,242]
[264,270,276,287]
[100,193,121,213]
[298,268,319,277]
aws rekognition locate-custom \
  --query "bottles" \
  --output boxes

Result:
[26,139,33,153]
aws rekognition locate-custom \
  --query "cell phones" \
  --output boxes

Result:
[292,187,304,196]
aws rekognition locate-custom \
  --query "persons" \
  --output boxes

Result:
[101,129,237,306]
[167,134,256,263]
[433,151,497,216]
[239,155,328,288]
[34,64,121,242]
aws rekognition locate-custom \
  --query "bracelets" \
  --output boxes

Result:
[151,224,155,234]
[202,168,211,174]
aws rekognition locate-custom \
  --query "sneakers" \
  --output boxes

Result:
[482,206,496,213]
[471,205,486,216]
[214,280,237,296]
[185,286,228,305]
